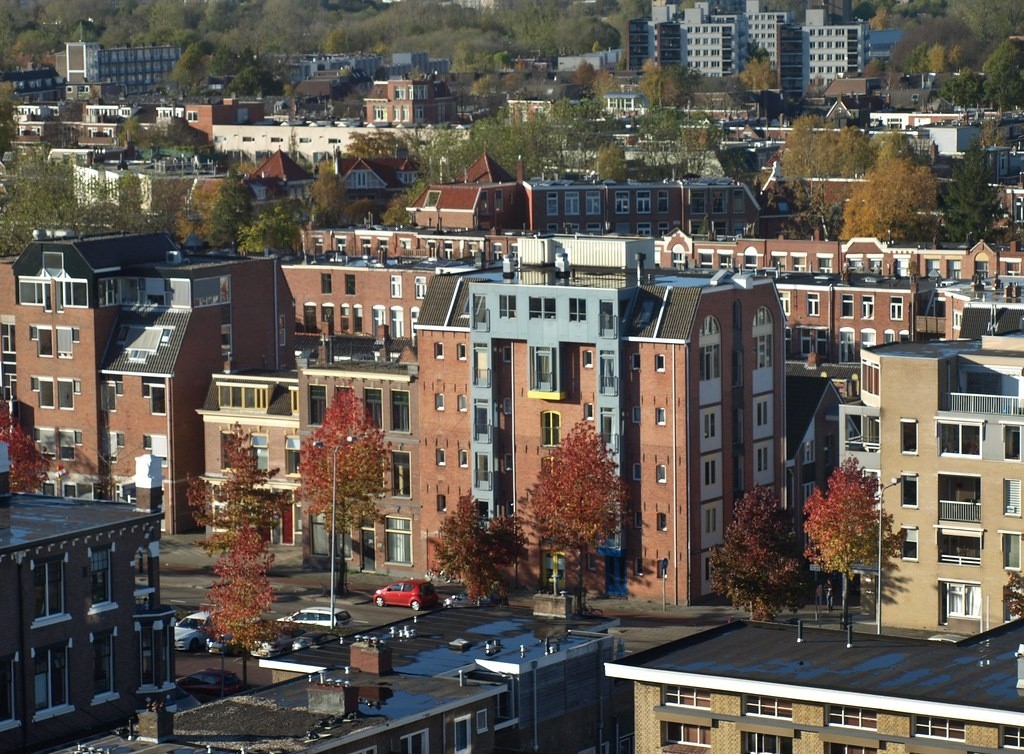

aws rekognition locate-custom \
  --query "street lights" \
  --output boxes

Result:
[313,436,356,630]
[878,478,904,635]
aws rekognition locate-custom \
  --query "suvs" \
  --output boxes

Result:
[276,607,355,629]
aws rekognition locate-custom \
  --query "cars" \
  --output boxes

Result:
[250,628,307,659]
[176,669,243,696]
[174,611,212,653]
[292,631,341,651]
[373,579,439,611]
[205,617,262,654]
[442,590,494,608]
